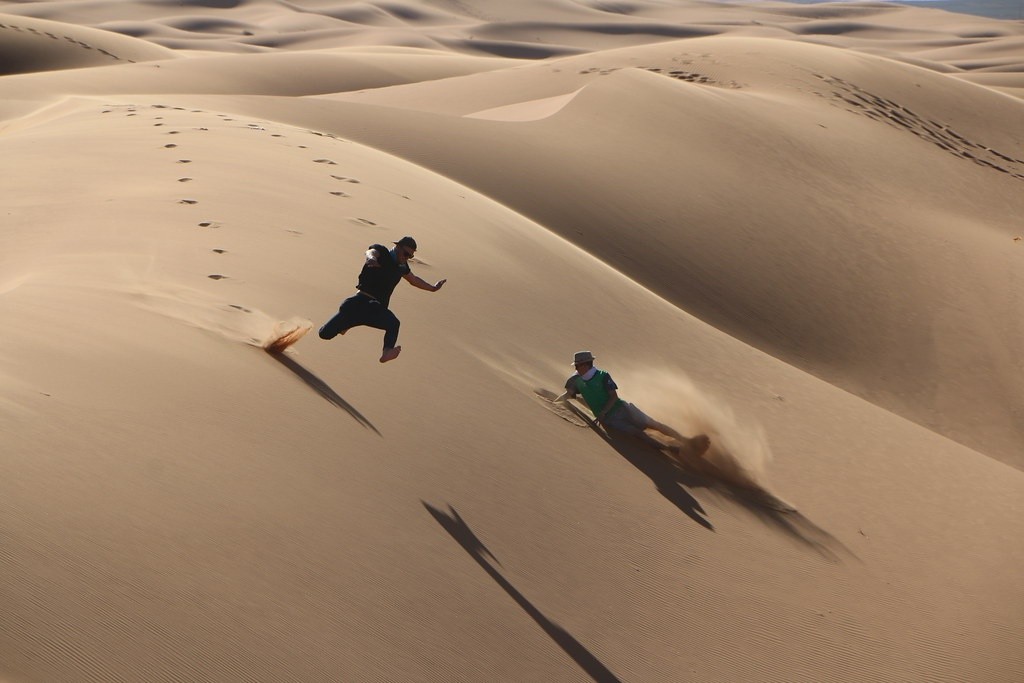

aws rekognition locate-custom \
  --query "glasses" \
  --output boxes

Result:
[402,248,414,259]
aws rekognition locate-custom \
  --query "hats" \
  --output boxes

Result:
[571,351,596,366]
[392,236,418,252]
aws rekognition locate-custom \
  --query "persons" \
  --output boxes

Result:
[554,351,693,448]
[318,236,447,363]
